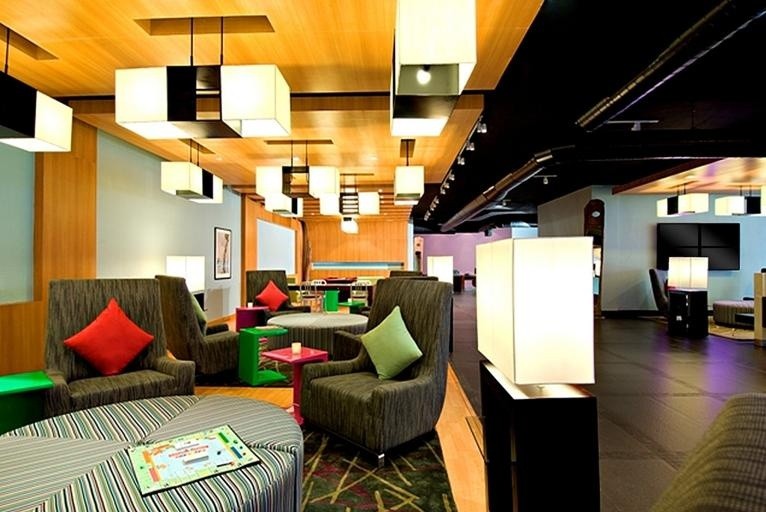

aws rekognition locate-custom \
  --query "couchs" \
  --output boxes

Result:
[714,301,754,327]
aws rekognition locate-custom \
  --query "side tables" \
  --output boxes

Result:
[0,370,54,435]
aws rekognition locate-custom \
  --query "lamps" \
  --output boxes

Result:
[161,140,223,204]
[256,142,379,234]
[393,139,425,205]
[0,28,72,153]
[669,257,708,339]
[475,237,601,512]
[656,185,766,218]
[113,17,290,140]
[389,0,477,137]
[423,115,489,221]
[167,257,205,310]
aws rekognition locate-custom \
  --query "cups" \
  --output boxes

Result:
[355,282,362,294]
[292,342,301,354]
[325,290,340,312]
[248,302,253,308]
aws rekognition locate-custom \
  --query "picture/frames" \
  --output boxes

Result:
[214,227,232,279]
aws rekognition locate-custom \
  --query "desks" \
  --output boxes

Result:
[0,394,305,512]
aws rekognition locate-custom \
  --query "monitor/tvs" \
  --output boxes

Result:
[656,222,740,271]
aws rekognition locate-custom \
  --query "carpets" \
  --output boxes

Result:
[708,316,754,340]
[301,423,457,512]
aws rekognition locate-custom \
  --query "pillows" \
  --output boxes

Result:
[65,298,153,375]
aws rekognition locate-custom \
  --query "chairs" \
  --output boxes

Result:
[650,268,668,318]
[648,392,766,512]
[43,278,195,418]
